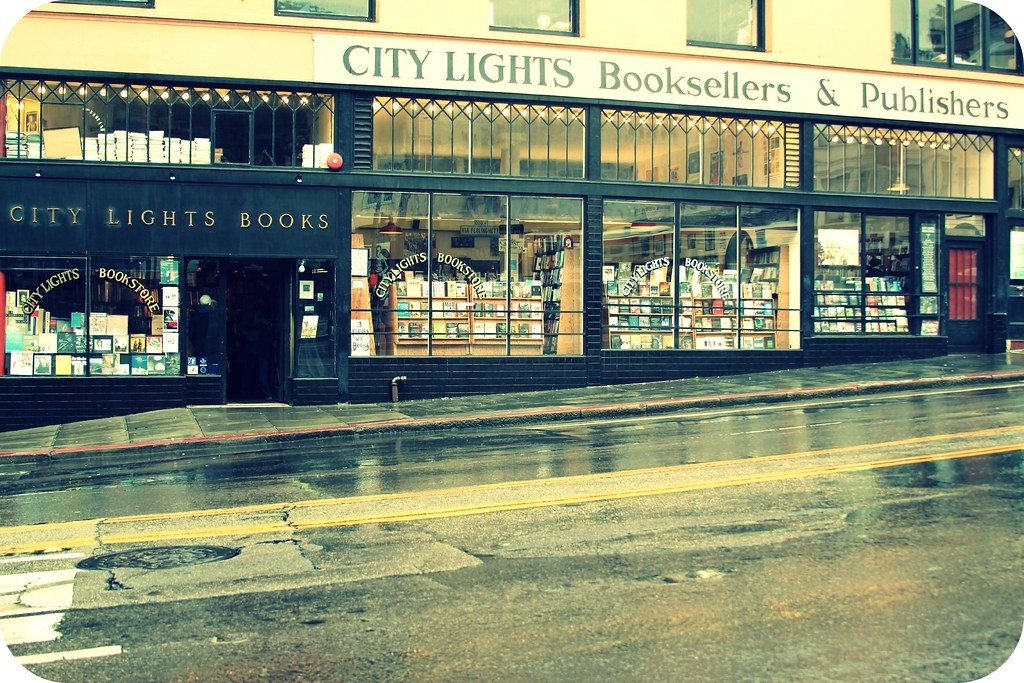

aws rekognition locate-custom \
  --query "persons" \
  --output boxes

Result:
[866,258,883,277]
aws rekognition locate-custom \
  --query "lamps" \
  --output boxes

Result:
[630,208,658,230]
[296,173,303,183]
[169,169,176,180]
[886,142,910,192]
[378,215,404,235]
[34,166,42,177]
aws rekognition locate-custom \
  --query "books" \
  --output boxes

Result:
[6,130,211,165]
[301,143,333,168]
[814,239,910,332]
[603,250,780,350]
[396,231,580,354]
[5,260,180,376]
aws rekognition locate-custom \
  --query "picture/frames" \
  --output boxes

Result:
[704,227,715,250]
[653,234,666,256]
[359,192,396,210]
[24,111,39,133]
[640,237,650,257]
[671,233,681,254]
[632,237,640,256]
[687,234,696,250]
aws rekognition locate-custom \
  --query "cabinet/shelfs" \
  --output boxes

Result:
[468,283,544,355]
[693,282,777,348]
[4,256,217,376]
[530,246,580,355]
[602,282,695,349]
[814,231,914,335]
[748,244,790,348]
[680,253,725,274]
[388,280,472,354]
